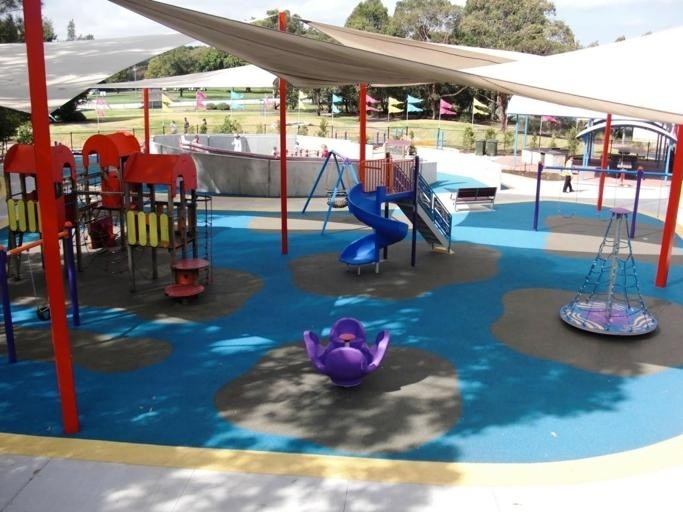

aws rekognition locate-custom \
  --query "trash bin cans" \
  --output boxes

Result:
[476,139,497,157]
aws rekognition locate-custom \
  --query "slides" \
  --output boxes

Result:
[338,183,413,264]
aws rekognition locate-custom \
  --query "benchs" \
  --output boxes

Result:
[447,185,498,213]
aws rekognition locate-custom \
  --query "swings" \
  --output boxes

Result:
[558,167,578,219]
[597,173,618,221]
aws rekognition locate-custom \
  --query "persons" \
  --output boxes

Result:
[231,135,242,152]
[199,118,207,134]
[562,156,574,192]
[171,120,177,134]
[183,116,189,133]
[192,135,199,143]
[273,139,329,158]
